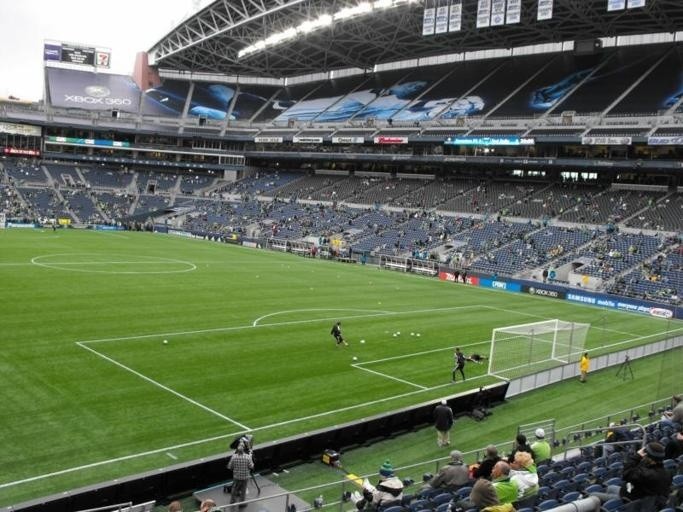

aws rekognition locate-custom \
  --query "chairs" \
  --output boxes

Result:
[378,418,683,512]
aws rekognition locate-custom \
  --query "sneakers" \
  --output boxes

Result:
[437,441,449,447]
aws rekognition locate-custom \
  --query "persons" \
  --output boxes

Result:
[463,353,488,365]
[227,442,254,508]
[168,501,183,512]
[1,142,683,306]
[580,352,590,383]
[352,393,683,512]
[451,348,466,384]
[200,499,222,512]
[331,322,344,347]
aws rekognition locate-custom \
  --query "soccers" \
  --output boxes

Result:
[164,340,168,344]
[417,333,420,336]
[411,333,414,336]
[361,340,365,343]
[353,357,357,361]
[393,331,401,337]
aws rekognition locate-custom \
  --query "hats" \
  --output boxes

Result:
[380,460,394,475]
[646,442,665,459]
[535,427,544,437]
[514,451,536,473]
[471,478,498,507]
[442,400,447,404]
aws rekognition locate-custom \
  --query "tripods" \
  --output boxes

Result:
[249,469,260,493]
[616,354,634,381]
